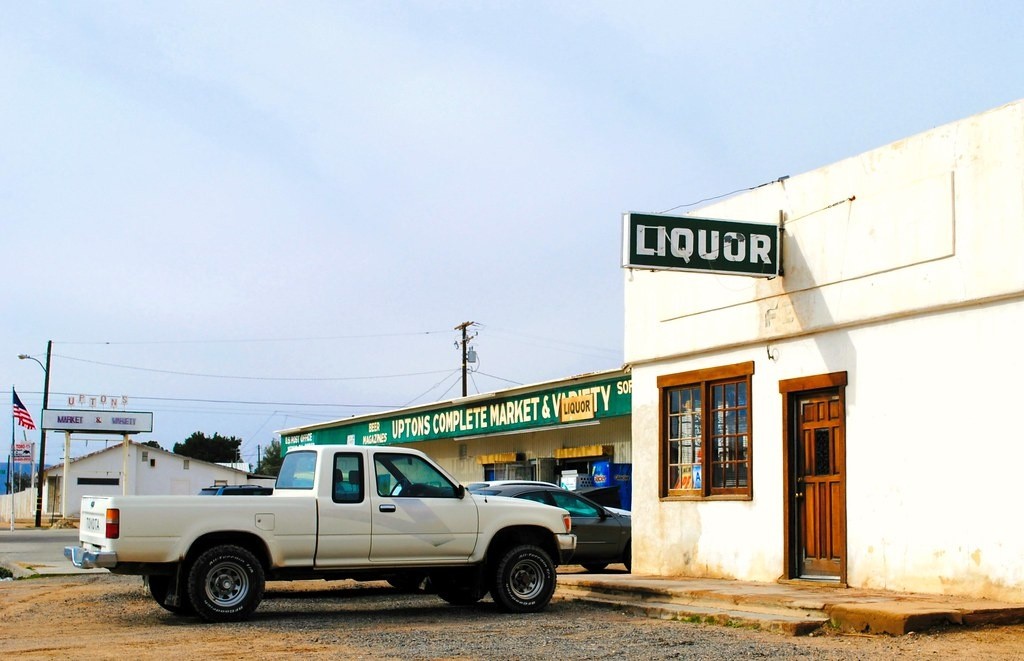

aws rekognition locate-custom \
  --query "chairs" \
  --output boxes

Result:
[346,470,359,501]
[334,469,346,500]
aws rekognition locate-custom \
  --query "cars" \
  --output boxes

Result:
[460,479,631,575]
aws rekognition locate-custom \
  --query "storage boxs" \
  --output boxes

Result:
[592,462,631,487]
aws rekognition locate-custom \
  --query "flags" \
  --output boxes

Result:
[13,388,36,431]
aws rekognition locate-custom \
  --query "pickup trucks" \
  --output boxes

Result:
[60,442,576,623]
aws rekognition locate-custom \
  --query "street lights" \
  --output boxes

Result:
[17,339,52,527]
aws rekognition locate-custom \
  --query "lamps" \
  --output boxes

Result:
[150,459,155,466]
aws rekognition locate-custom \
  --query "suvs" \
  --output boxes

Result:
[192,483,273,496]
